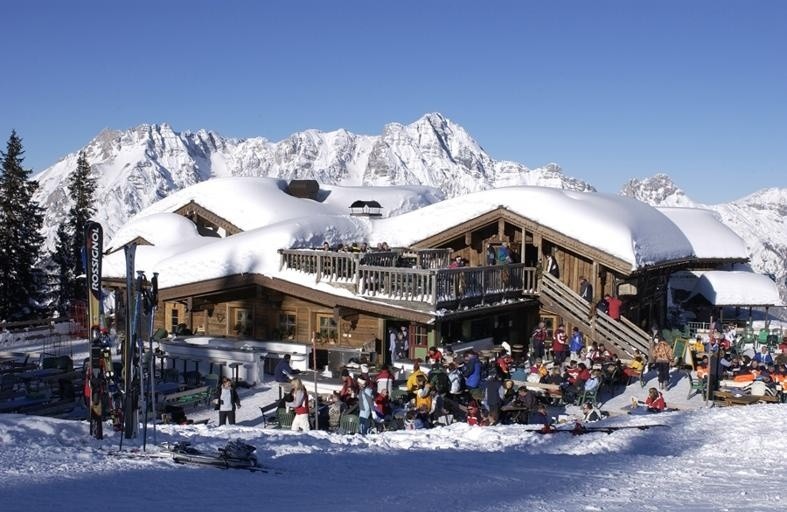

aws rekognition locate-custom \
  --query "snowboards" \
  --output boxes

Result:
[101,335,123,431]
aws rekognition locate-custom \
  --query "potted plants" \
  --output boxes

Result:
[274,325,338,346]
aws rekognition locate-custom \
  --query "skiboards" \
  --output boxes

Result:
[99,448,285,475]
[124,242,139,440]
[84,220,102,440]
[526,424,669,433]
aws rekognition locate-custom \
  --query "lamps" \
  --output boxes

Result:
[183,303,189,314]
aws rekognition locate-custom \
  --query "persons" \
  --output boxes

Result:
[594,294,610,337]
[498,240,512,265]
[337,321,787,435]
[213,376,241,426]
[285,377,310,431]
[607,296,622,345]
[577,276,593,320]
[444,256,462,283]
[321,242,391,278]
[460,260,485,294]
[274,354,300,383]
[484,240,496,273]
[542,252,560,296]
[51,306,59,324]
[276,388,295,413]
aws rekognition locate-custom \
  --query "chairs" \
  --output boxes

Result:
[338,413,360,436]
[273,408,294,431]
[663,314,787,353]
[477,343,759,427]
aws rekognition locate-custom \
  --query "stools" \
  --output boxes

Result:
[214,360,226,380]
[232,361,242,383]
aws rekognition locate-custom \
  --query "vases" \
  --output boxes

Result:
[232,329,239,337]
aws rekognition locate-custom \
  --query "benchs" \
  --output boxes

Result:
[0,351,218,423]
[257,399,285,429]
